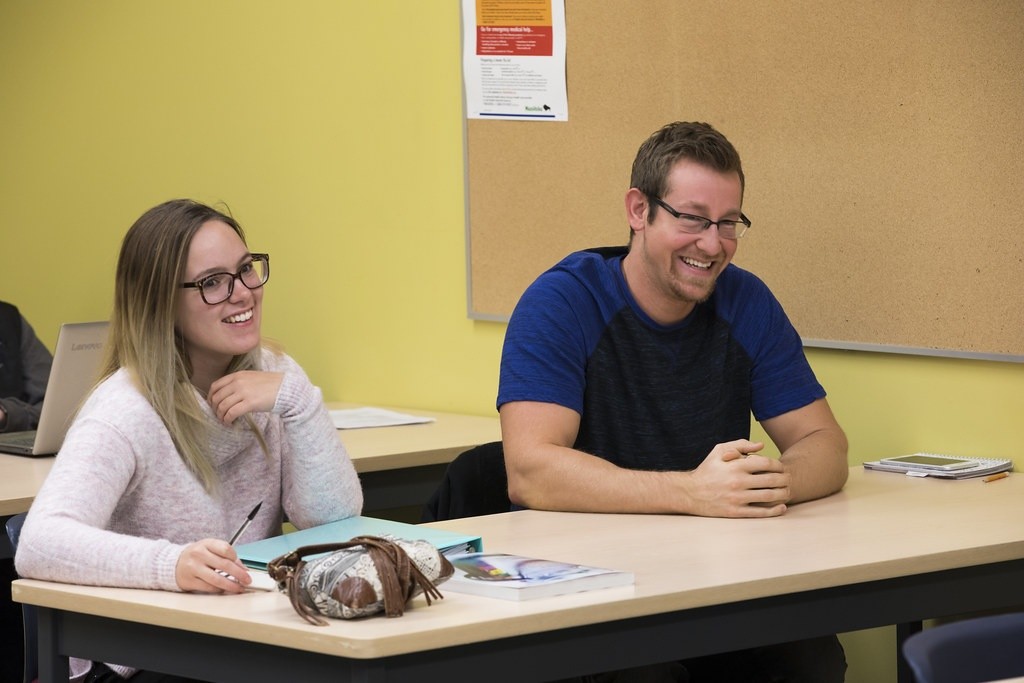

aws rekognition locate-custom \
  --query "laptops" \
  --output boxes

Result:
[0,322,111,457]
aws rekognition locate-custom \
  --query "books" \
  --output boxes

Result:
[864,452,1013,480]
[422,553,635,601]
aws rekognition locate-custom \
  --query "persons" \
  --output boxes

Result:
[15,199,363,683]
[0,301,53,683]
[496,122,848,683]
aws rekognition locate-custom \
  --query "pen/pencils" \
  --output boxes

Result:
[982,472,1009,483]
[229,500,263,545]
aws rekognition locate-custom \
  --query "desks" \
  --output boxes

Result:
[9,461,1024,683]
[0,406,501,516]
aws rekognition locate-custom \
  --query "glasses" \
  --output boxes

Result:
[177,253,270,305]
[644,190,751,239]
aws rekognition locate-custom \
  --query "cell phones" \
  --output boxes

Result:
[881,455,980,471]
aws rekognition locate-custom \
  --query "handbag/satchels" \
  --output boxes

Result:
[268,531,455,627]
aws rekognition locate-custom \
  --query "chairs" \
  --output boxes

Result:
[902,610,1024,682]
[423,438,511,523]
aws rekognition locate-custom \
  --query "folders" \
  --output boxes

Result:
[233,515,483,571]
